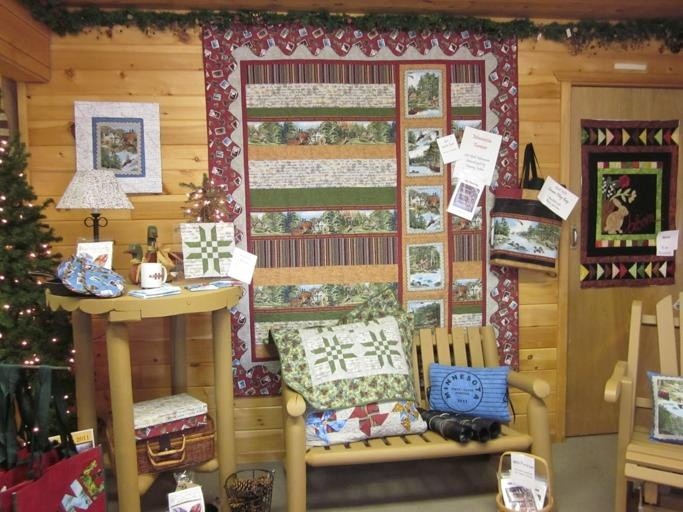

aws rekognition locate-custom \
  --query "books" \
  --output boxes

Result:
[497,454,548,511]
[47,427,95,460]
[165,485,204,512]
[128,281,182,299]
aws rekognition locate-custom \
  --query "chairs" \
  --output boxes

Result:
[265,318,559,512]
[600,289,683,512]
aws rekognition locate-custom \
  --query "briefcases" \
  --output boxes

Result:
[105,416,216,474]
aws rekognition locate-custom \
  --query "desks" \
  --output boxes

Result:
[44,274,247,510]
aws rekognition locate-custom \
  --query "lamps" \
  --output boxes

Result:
[52,167,137,270]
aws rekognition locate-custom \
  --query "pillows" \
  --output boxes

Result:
[301,400,430,453]
[424,357,514,427]
[270,314,418,411]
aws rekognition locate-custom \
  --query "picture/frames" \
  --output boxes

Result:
[71,97,165,196]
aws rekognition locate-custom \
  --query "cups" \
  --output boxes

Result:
[140,262,168,289]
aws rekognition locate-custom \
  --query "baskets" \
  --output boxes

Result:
[496,451,553,512]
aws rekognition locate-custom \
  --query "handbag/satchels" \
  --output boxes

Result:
[1,440,106,512]
[491,186,562,274]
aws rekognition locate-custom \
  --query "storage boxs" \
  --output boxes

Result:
[130,389,210,443]
[133,412,216,477]
